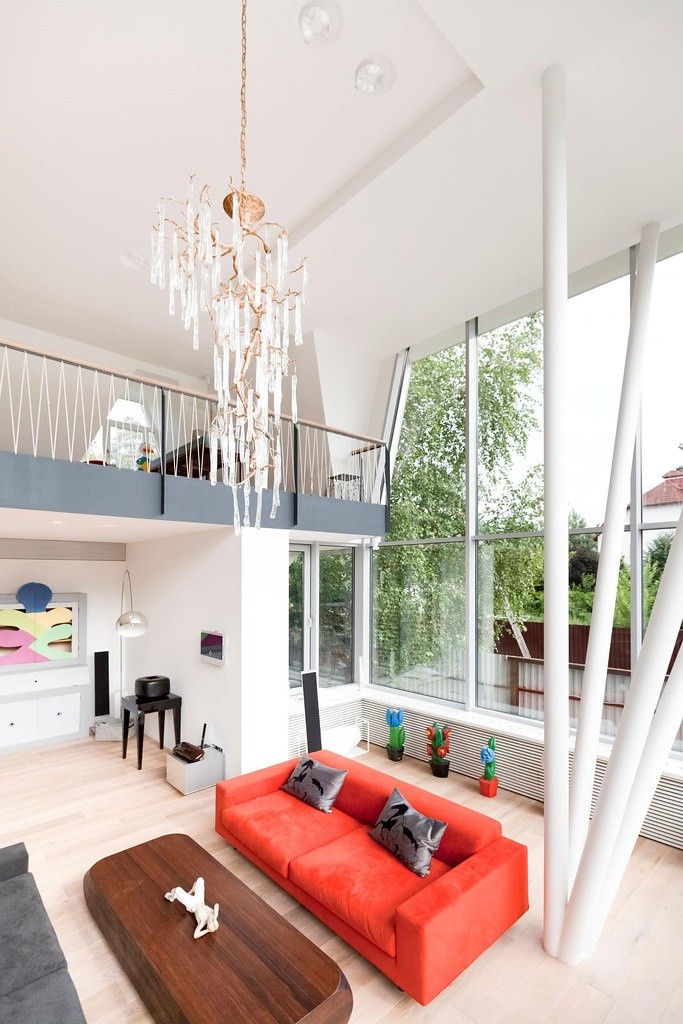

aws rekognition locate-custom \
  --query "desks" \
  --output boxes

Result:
[150,434,254,483]
[121,692,182,769]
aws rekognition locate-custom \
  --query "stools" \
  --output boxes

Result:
[324,473,364,503]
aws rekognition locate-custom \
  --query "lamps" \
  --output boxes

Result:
[150,0,308,538]
[115,569,150,726]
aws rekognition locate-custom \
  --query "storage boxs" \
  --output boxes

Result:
[166,747,225,795]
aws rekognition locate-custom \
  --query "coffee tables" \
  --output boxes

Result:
[83,833,353,1024]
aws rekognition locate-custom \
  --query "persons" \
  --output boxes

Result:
[164,877,220,939]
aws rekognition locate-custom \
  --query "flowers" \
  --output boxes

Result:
[386,705,406,749]
[426,721,452,762]
[480,735,497,780]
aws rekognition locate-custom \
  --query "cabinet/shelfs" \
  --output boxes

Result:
[0,683,90,756]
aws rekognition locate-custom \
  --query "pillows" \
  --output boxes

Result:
[280,752,348,813]
[368,787,448,878]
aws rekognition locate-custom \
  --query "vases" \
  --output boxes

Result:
[429,759,450,778]
[479,775,499,798]
[386,744,405,761]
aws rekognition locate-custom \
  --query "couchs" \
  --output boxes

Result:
[218,749,531,1006]
[0,842,88,1024]
